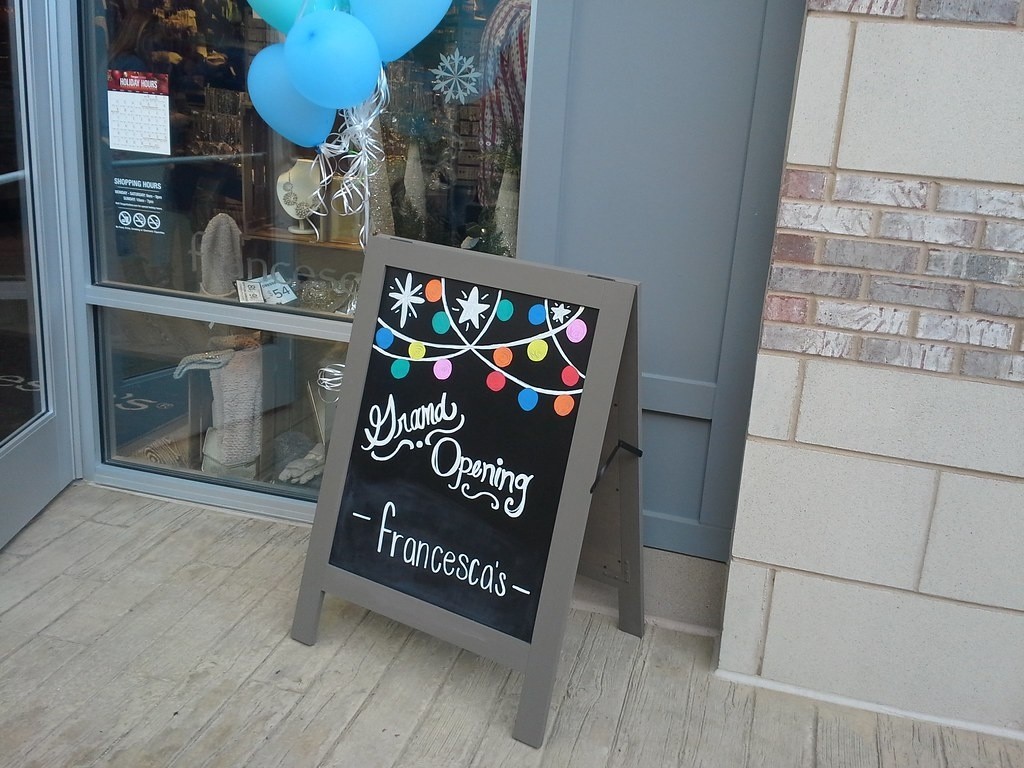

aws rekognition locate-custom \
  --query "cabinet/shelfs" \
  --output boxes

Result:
[187,95,369,491]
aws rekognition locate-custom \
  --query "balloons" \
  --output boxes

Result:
[246,0,453,148]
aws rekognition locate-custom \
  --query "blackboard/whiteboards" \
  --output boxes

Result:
[287,232,638,675]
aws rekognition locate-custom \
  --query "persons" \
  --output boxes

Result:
[107,7,158,73]
[478,0,531,206]
